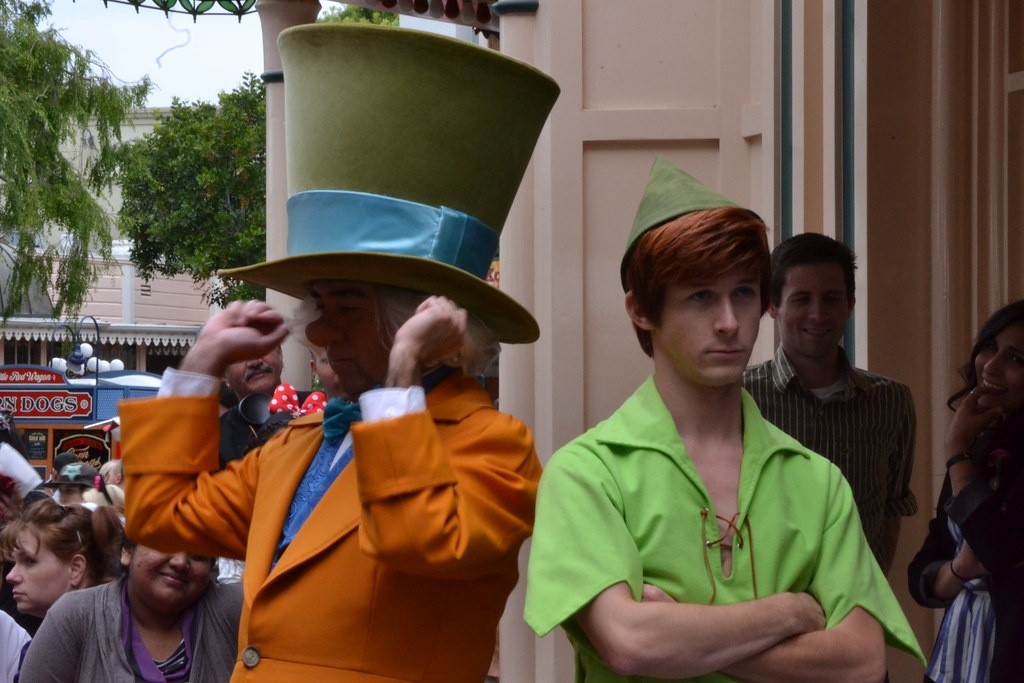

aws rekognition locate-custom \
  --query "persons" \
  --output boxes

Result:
[522,154,928,683]
[116,190,544,683]
[908,299,1024,683]
[0,344,349,683]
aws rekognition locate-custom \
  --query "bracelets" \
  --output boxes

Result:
[946,451,973,473]
[950,560,971,581]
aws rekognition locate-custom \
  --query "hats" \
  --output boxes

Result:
[618,154,766,292]
[43,462,97,488]
[216,24,561,344]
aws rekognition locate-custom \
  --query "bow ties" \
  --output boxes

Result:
[323,383,381,437]
